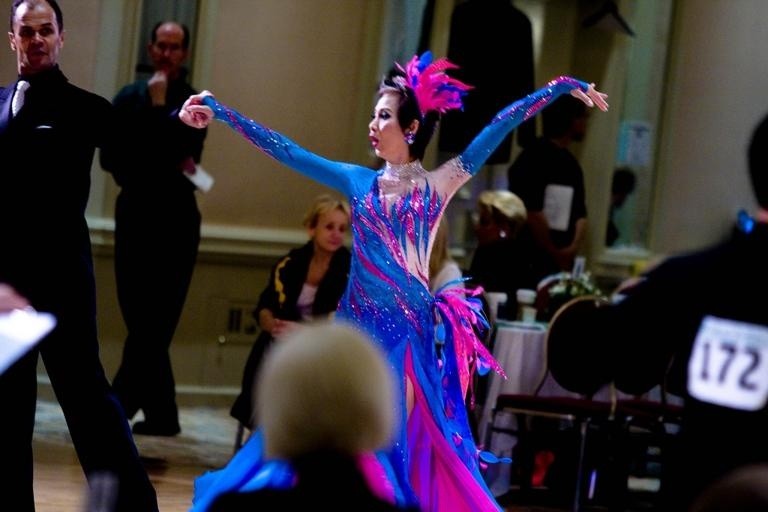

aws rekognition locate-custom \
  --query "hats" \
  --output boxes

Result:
[480,190,527,223]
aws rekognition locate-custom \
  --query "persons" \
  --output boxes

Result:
[547,116,767,511]
[0,283,33,315]
[0,2,212,510]
[94,20,207,435]
[465,189,556,323]
[204,318,410,509]
[505,85,588,261]
[228,194,351,438]
[182,43,613,510]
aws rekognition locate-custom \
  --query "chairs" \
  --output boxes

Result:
[482,295,687,512]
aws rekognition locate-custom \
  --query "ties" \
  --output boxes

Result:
[11,81,30,117]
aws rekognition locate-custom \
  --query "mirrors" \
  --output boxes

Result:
[594,0,678,270]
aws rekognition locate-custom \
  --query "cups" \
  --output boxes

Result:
[516,288,537,323]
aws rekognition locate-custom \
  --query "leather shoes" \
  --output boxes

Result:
[132,422,182,437]
